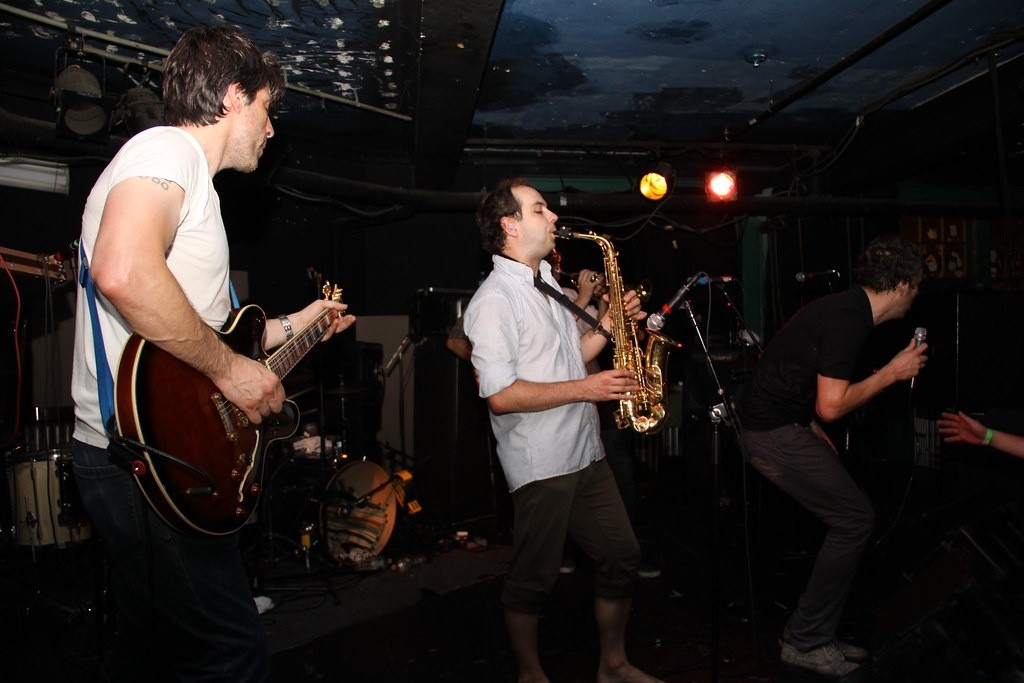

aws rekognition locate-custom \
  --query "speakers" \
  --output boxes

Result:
[839,500,1024,683]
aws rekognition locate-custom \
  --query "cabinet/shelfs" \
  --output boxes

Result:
[899,215,967,278]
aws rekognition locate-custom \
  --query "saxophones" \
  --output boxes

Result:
[553,227,682,438]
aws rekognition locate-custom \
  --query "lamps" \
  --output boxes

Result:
[702,142,743,201]
[637,142,678,201]
[40,31,117,146]
[119,62,166,135]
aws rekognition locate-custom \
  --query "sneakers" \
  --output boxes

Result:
[781,641,860,678]
[830,640,868,659]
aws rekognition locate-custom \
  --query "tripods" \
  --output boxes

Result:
[240,443,335,571]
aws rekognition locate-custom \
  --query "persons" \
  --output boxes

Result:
[734,233,927,676]
[446,176,669,683]
[72,26,356,683]
[937,410,1024,458]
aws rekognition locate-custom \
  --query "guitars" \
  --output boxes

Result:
[114,283,347,540]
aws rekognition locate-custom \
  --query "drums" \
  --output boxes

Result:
[4,446,98,554]
[261,449,396,568]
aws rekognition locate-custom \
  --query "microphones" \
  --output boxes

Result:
[334,505,351,519]
[796,269,836,282]
[910,327,927,392]
[646,272,703,332]
[386,335,412,370]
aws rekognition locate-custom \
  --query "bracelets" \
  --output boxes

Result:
[982,427,993,446]
[279,315,294,340]
